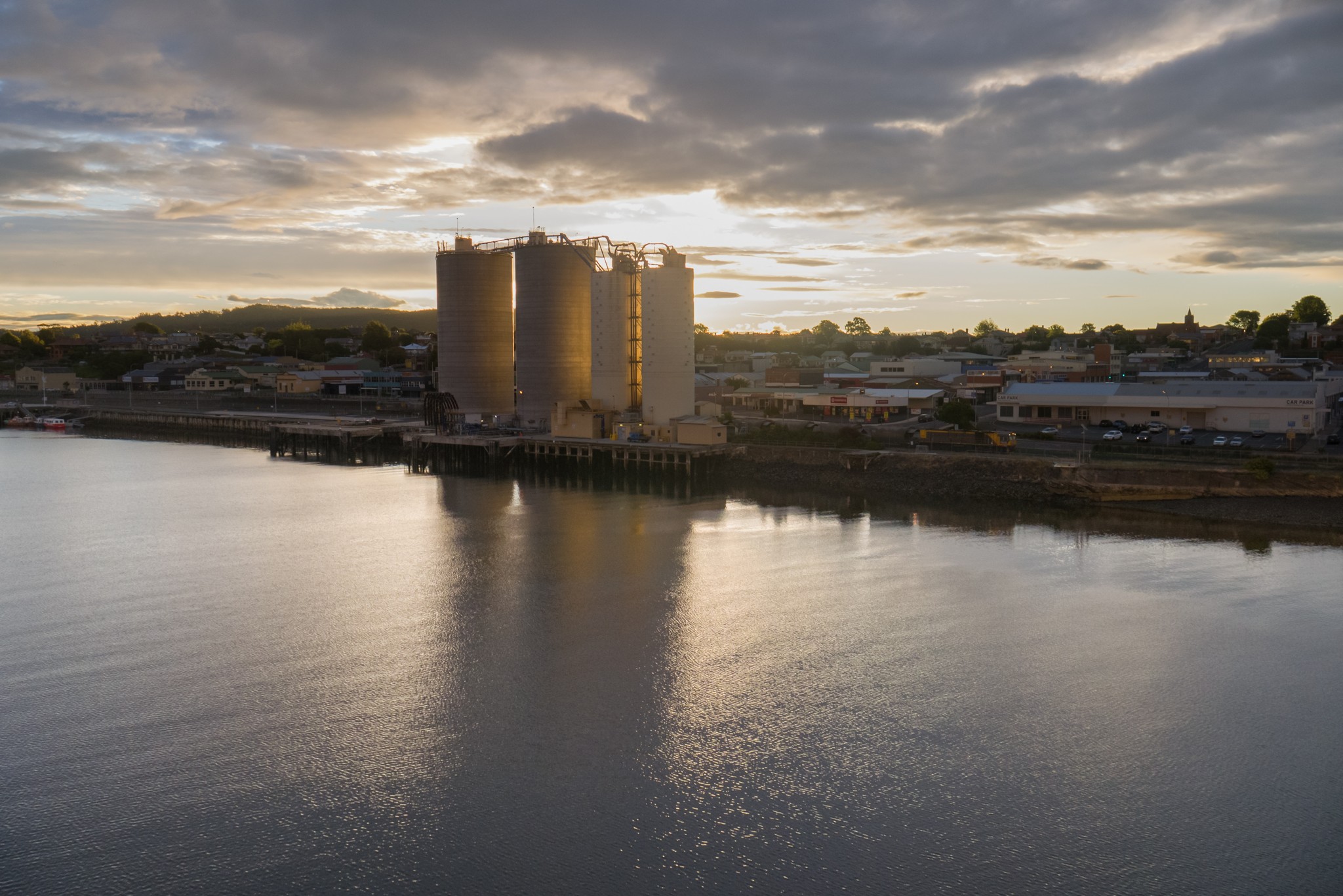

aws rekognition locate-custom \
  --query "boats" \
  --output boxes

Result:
[4,416,85,429]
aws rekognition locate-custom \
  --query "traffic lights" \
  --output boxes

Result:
[1109,373,1113,380]
[1122,372,1125,377]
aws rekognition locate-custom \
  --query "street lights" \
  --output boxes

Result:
[651,407,653,425]
[715,388,725,419]
[1081,423,1088,464]
[15,363,23,391]
[130,364,138,392]
[612,395,615,417]
[1164,391,1169,448]
[782,372,797,419]
[907,382,918,421]
[960,382,976,428]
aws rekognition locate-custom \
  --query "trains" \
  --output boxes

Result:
[721,420,1017,453]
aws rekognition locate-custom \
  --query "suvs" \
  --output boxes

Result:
[918,413,934,423]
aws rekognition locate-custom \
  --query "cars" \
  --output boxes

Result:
[1231,437,1245,445]
[1098,419,1127,428]
[1327,434,1340,444]
[1213,436,1227,446]
[1149,424,1164,433]
[1040,427,1059,436]
[1181,434,1194,444]
[1132,423,1147,434]
[1180,425,1193,434]
[1137,431,1150,440]
[1103,430,1123,440]
[1253,430,1266,438]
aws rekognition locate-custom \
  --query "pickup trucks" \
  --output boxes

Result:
[626,433,652,443]
[366,417,386,424]
